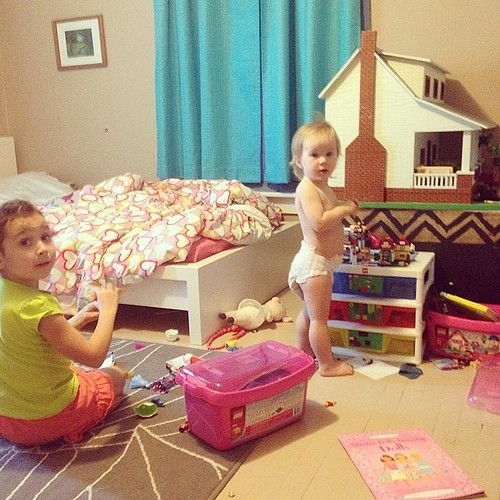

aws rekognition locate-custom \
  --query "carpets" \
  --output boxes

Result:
[0,327,265,500]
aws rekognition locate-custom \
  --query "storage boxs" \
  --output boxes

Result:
[176,340,318,451]
[429,303,500,361]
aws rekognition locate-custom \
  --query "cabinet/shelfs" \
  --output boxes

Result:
[326,252,435,366]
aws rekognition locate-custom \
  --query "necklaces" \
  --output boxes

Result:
[208,324,245,351]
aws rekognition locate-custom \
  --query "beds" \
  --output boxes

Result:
[1,136,302,347]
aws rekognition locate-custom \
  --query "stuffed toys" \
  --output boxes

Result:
[219,296,293,330]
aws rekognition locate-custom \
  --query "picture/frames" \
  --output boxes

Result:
[52,15,108,73]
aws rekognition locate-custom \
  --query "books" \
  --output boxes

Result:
[338,429,485,500]
[165,353,204,370]
[440,291,499,322]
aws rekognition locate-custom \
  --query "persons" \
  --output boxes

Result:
[289,122,357,377]
[0,198,125,445]
